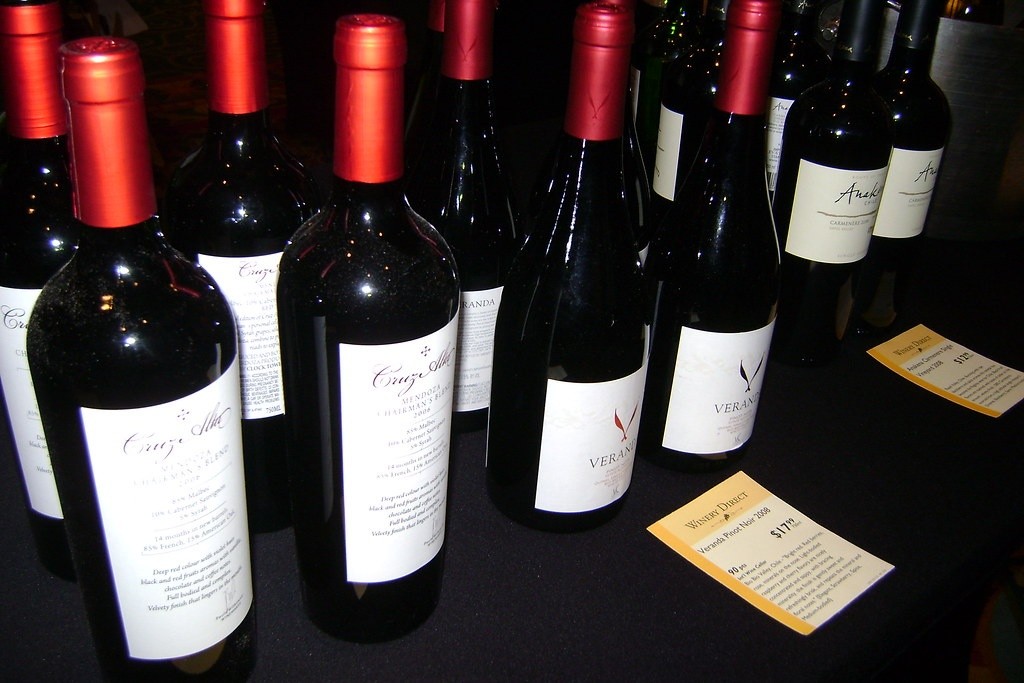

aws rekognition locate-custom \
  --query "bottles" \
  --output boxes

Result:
[0,0,949,683]
